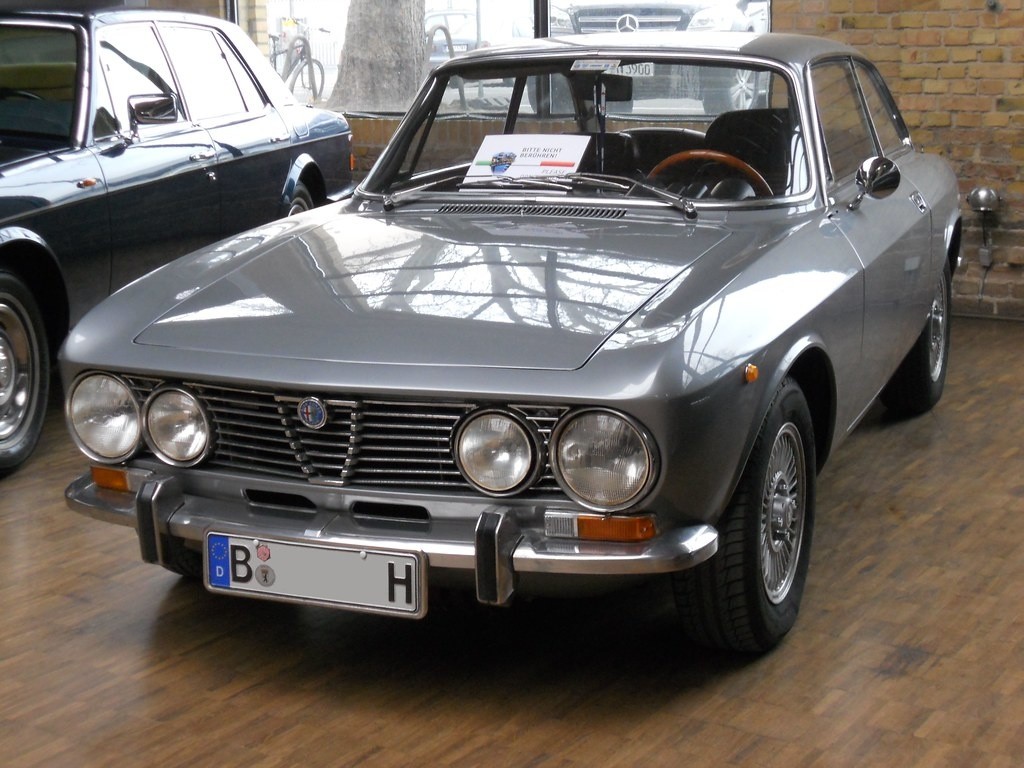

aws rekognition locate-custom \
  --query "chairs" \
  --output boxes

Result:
[559,105,797,196]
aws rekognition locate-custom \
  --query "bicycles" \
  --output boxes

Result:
[267,16,331,104]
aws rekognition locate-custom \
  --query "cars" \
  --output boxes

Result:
[57,30,967,657]
[423,8,517,86]
[523,0,771,118]
[0,11,356,490]
